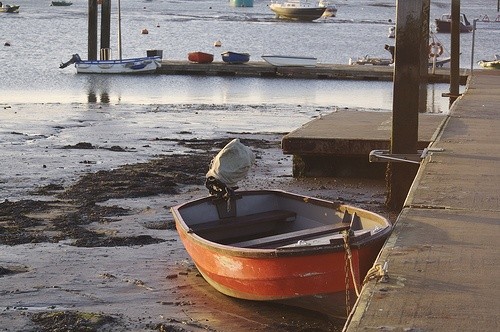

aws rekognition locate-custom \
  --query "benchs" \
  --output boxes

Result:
[228,222,350,249]
[188,209,297,236]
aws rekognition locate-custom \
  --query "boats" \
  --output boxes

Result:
[59,53,162,74]
[220,51,251,64]
[434,13,473,33]
[349,22,462,67]
[261,55,317,67]
[0,1,21,13]
[267,0,328,21]
[49,0,73,6]
[228,0,255,7]
[187,51,214,64]
[319,0,337,17]
[477,54,500,70]
[170,176,393,301]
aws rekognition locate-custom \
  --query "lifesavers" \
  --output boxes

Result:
[428,42,443,57]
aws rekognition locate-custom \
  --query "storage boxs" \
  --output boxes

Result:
[147,50,162,59]
